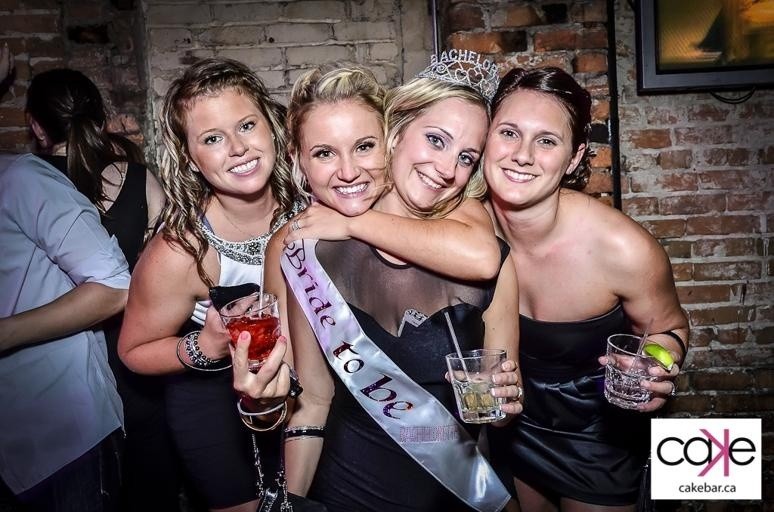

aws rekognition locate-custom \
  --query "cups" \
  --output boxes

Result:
[219,290,281,370]
[600,333,659,410]
[445,348,511,424]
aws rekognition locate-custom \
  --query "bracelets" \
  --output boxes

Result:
[174,326,233,374]
[280,422,328,442]
[662,329,687,370]
[234,395,288,433]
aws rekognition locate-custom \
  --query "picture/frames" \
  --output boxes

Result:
[629,0,774,98]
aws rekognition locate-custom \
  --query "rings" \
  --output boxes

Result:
[290,218,301,230]
[662,380,676,396]
[516,386,524,398]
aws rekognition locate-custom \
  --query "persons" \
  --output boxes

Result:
[477,63,693,512]
[0,150,130,512]
[22,66,168,271]
[114,51,310,512]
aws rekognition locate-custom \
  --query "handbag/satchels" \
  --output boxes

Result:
[257,488,327,511]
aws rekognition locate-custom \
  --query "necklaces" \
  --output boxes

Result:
[216,202,255,238]
